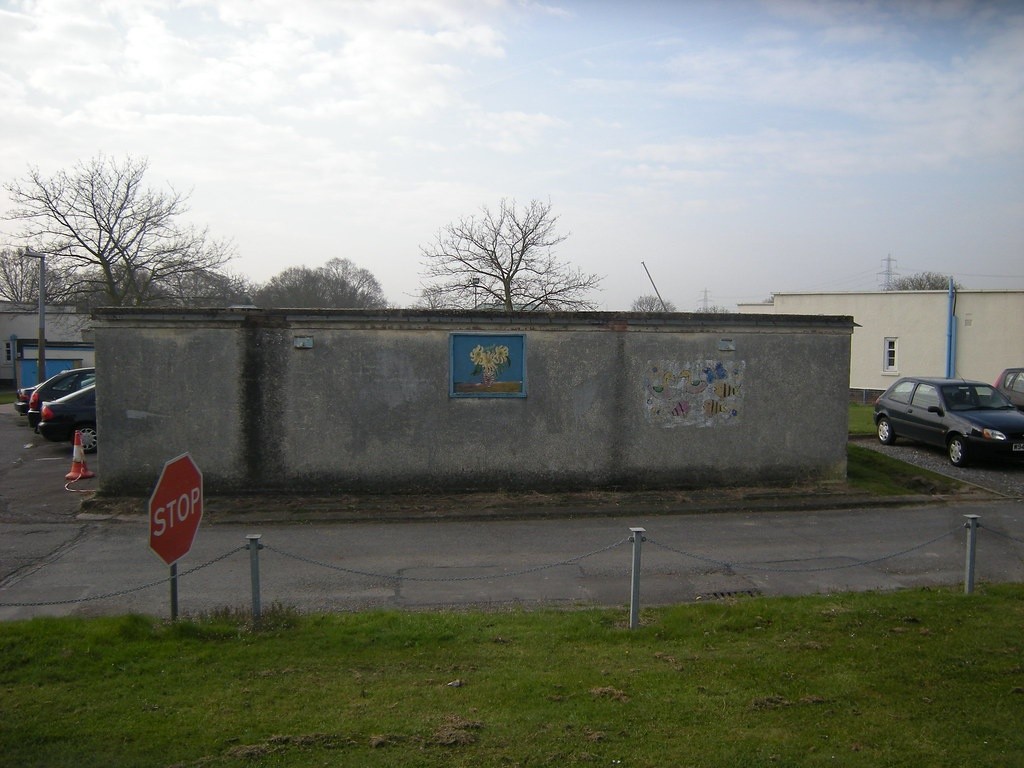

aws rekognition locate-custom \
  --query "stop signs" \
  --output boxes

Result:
[148,452,205,569]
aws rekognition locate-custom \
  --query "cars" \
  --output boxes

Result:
[40,384,97,454]
[872,377,1024,467]
[14,375,97,413]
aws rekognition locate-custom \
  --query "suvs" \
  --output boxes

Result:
[27,367,96,429]
[991,368,1024,410]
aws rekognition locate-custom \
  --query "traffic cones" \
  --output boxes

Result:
[64,430,96,480]
